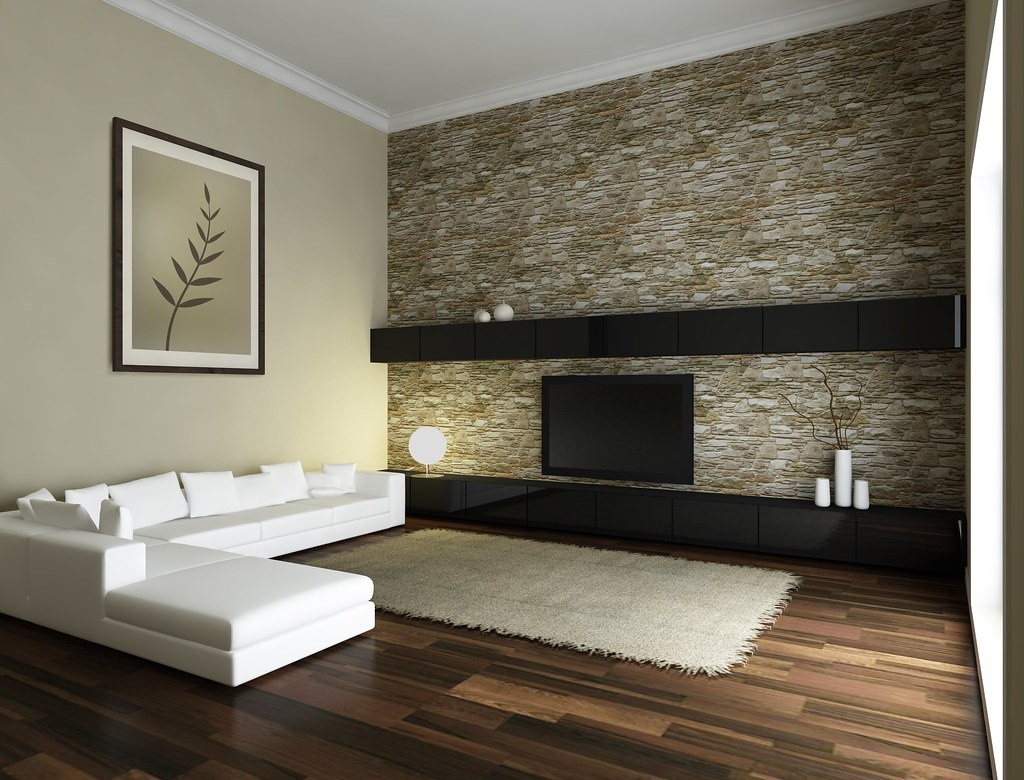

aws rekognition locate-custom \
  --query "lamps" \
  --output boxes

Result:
[408,426,447,478]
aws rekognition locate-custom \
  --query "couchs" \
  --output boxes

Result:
[0,471,406,686]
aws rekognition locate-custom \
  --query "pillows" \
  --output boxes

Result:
[30,500,97,533]
[17,487,57,521]
[234,472,286,510]
[108,471,190,531]
[98,499,133,540]
[310,487,347,497]
[322,463,357,493]
[180,471,239,518]
[65,483,108,529]
[260,460,313,503]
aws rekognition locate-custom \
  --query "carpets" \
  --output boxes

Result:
[305,530,803,679]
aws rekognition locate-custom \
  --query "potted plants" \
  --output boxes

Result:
[778,365,863,508]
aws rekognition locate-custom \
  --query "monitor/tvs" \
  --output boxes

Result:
[542,374,694,484]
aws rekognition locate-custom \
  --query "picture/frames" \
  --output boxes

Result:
[112,114,266,375]
